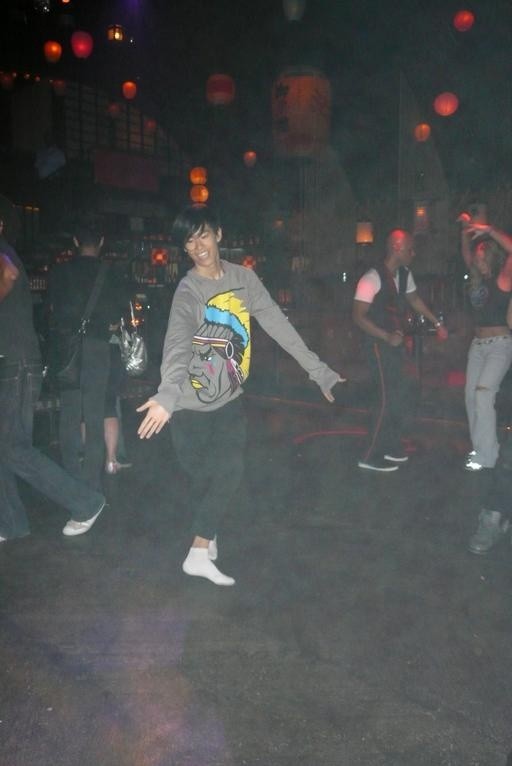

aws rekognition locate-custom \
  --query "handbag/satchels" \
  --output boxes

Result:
[120,302,148,376]
[47,331,83,387]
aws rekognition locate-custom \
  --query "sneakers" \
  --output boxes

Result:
[384,451,408,464]
[118,458,133,468]
[464,448,494,471]
[357,456,399,474]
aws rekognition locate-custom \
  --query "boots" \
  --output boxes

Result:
[467,507,509,554]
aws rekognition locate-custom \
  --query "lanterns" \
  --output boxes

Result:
[356,222,374,246]
[433,92,459,116]
[243,151,256,167]
[107,103,120,116]
[283,0,306,22]
[44,40,62,62]
[71,31,93,58]
[453,10,475,32]
[0,71,66,90]
[414,123,431,141]
[144,119,156,132]
[122,81,137,99]
[190,166,209,208]
[107,24,123,42]
[206,74,235,109]
[271,64,332,165]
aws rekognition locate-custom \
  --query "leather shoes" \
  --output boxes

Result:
[105,461,118,475]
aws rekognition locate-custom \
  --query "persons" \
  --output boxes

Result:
[456,212,512,472]
[0,219,133,544]
[189,292,251,403]
[352,228,448,471]
[136,208,346,585]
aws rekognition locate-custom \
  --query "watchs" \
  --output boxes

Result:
[434,321,443,328]
